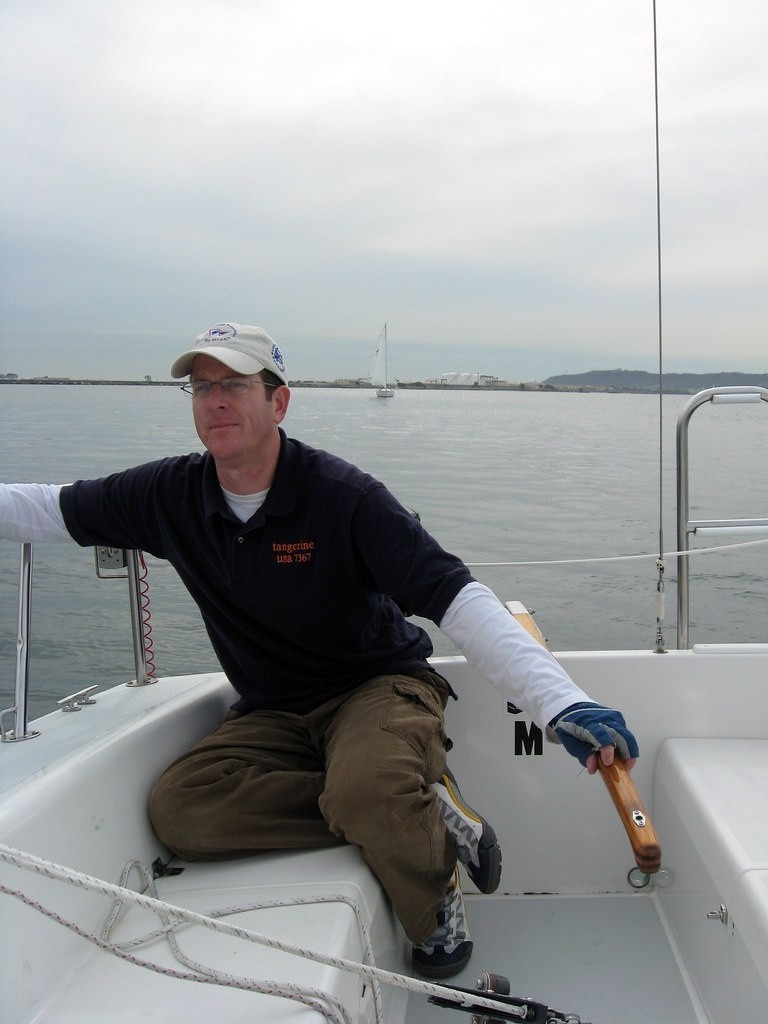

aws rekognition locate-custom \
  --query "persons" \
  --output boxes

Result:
[0,323,640,981]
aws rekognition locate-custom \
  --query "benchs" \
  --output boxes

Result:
[28,843,405,1024]
[651,739,767,1024]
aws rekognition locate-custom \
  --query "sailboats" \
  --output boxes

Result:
[368,322,395,398]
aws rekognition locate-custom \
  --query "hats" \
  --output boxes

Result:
[170,322,289,386]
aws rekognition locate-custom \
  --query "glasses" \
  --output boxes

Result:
[180,376,276,399]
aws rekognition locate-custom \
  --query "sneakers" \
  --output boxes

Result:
[412,864,473,978]
[432,764,502,895]
